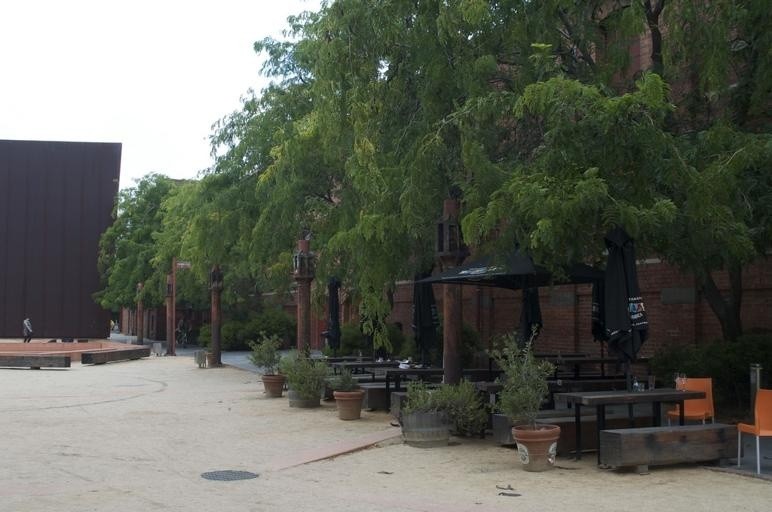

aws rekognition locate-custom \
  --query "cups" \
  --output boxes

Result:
[379,357,410,369]
[648,375,656,392]
[675,377,688,392]
[638,383,645,393]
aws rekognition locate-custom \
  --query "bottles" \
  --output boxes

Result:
[632,376,639,393]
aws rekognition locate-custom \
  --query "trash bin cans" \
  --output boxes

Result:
[194,350,206,368]
[153,342,162,356]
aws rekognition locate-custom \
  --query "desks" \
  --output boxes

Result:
[542,354,654,390]
[328,356,444,411]
[568,390,706,458]
[461,367,506,412]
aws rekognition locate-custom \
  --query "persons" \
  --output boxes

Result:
[175,314,192,344]
[23,317,33,343]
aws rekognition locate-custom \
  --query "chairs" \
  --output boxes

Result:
[552,392,677,418]
[673,378,715,425]
[491,407,666,453]
[324,375,445,421]
[736,389,772,474]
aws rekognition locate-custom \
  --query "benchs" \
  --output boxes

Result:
[81,347,150,365]
[599,423,744,475]
[0,356,71,370]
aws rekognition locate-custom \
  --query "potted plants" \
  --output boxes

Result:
[246,331,285,396]
[287,345,329,407]
[204,348,216,367]
[329,363,364,419]
[399,381,487,449]
[485,324,561,471]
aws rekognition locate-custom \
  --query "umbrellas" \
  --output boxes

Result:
[516,287,544,352]
[320,275,343,375]
[590,224,650,378]
[414,243,605,353]
[410,273,441,367]
